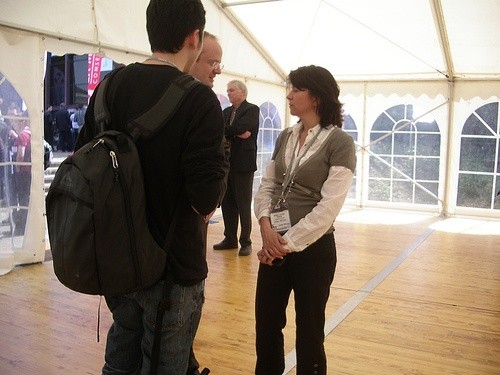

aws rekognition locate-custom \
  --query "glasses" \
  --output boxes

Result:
[210,60,224,71]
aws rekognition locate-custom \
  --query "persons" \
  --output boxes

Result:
[0,0,231,375]
[254,65,356,375]
[212,80,259,256]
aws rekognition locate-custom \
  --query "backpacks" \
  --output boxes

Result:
[23,128,53,172]
[46,67,201,375]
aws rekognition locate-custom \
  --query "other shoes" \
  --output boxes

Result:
[212,236,239,250]
[239,244,252,256]
[2,215,9,223]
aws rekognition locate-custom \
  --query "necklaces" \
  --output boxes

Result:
[281,144,306,208]
[144,57,179,69]
